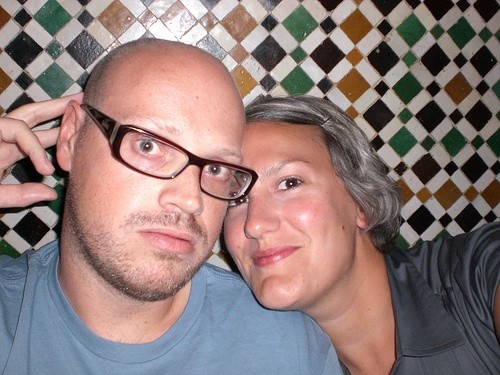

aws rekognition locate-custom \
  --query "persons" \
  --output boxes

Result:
[223,98,500,373]
[0,39,345,373]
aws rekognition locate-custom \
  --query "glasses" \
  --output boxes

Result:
[78,103,259,202]
